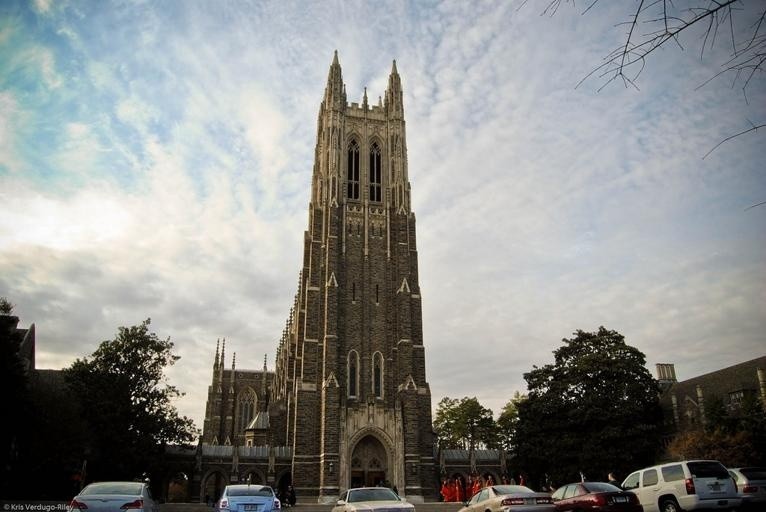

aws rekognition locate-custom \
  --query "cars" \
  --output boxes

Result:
[332,487,416,512]
[454,460,766,512]
[215,484,282,512]
[69,481,159,512]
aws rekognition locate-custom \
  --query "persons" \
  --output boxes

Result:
[608,471,621,488]
[284,484,297,507]
[438,474,526,502]
[542,470,555,492]
[273,485,282,506]
